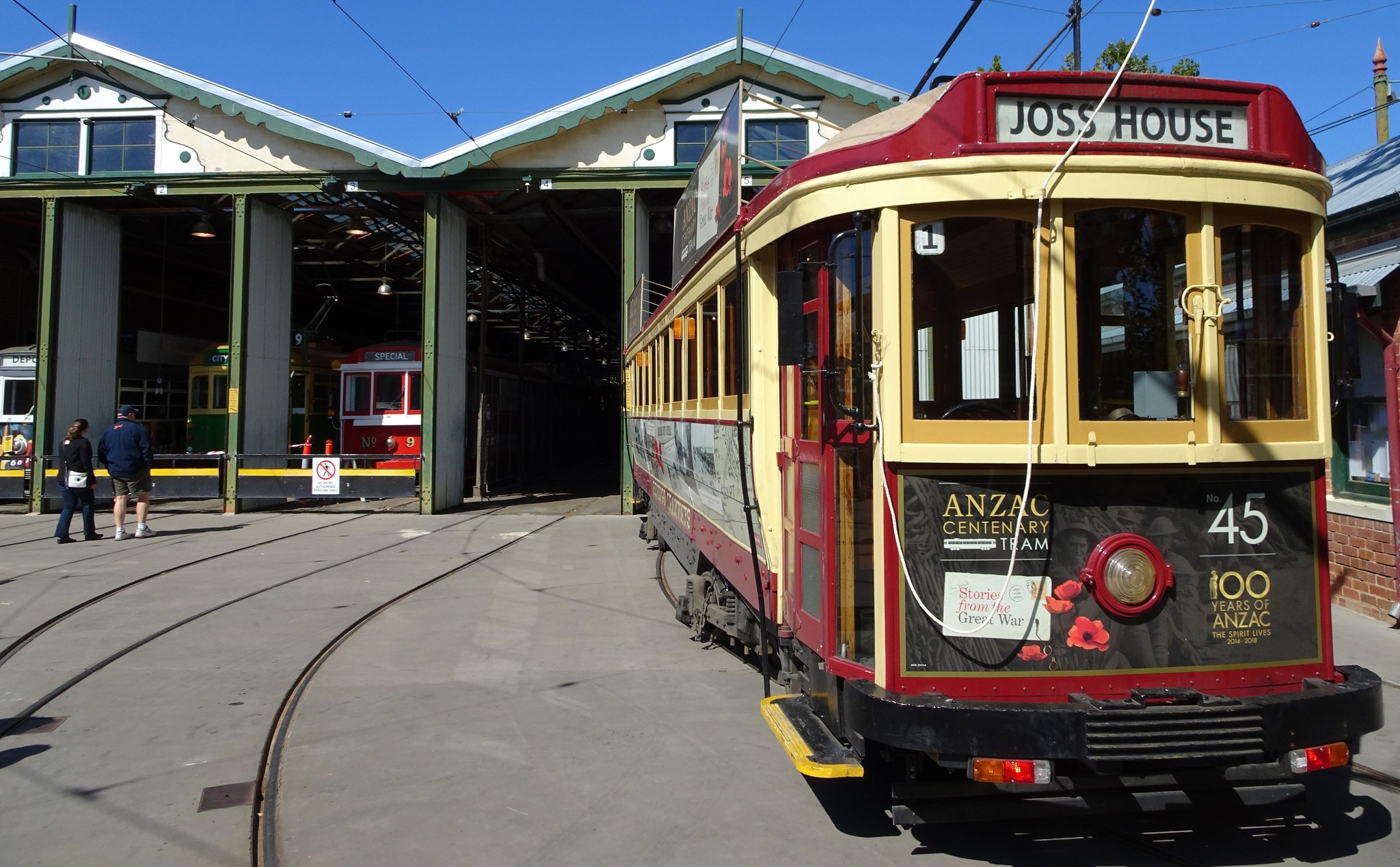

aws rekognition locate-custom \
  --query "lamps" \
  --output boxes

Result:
[524,333,531,340]
[602,361,607,364]
[377,282,393,295]
[190,215,216,237]
[467,314,478,322]
[561,346,568,351]
[345,213,369,234]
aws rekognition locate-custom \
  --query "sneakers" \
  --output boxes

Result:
[115,530,131,540]
[134,525,157,537]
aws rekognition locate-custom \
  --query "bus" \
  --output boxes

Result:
[185,342,340,456]
[620,68,1388,831]
[0,344,185,502]
[329,344,425,474]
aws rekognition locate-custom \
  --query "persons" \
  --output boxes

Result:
[97,405,157,541]
[54,418,104,544]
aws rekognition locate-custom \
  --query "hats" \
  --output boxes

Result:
[117,406,140,416]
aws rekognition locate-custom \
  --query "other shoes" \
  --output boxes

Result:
[57,536,77,544]
[84,532,103,541]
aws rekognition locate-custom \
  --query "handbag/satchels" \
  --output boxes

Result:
[65,469,88,490]
